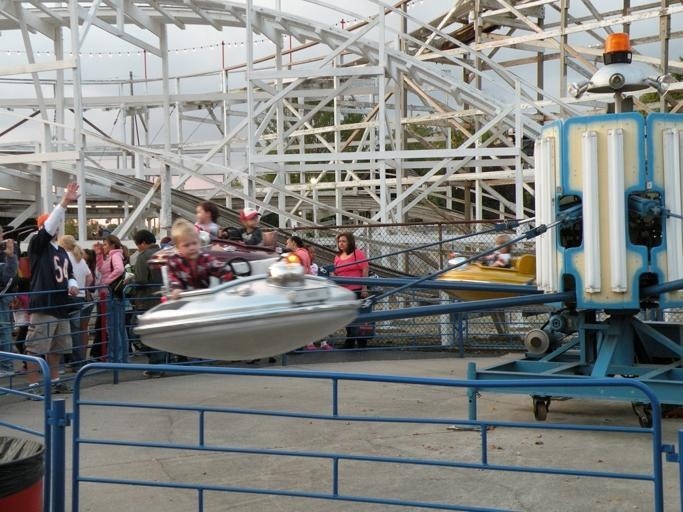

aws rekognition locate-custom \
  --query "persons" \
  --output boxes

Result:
[478,235,512,268]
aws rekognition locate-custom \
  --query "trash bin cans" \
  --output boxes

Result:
[0,436,45,512]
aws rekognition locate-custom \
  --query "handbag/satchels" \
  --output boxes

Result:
[366,270,384,293]
[350,296,377,340]
[107,256,126,303]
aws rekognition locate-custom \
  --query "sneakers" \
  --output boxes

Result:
[0,369,16,379]
[26,384,46,402]
[321,344,334,350]
[50,380,74,394]
[304,344,318,351]
[58,350,145,375]
[142,370,161,378]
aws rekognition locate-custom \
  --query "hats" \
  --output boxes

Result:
[37,213,49,227]
[240,207,261,221]
